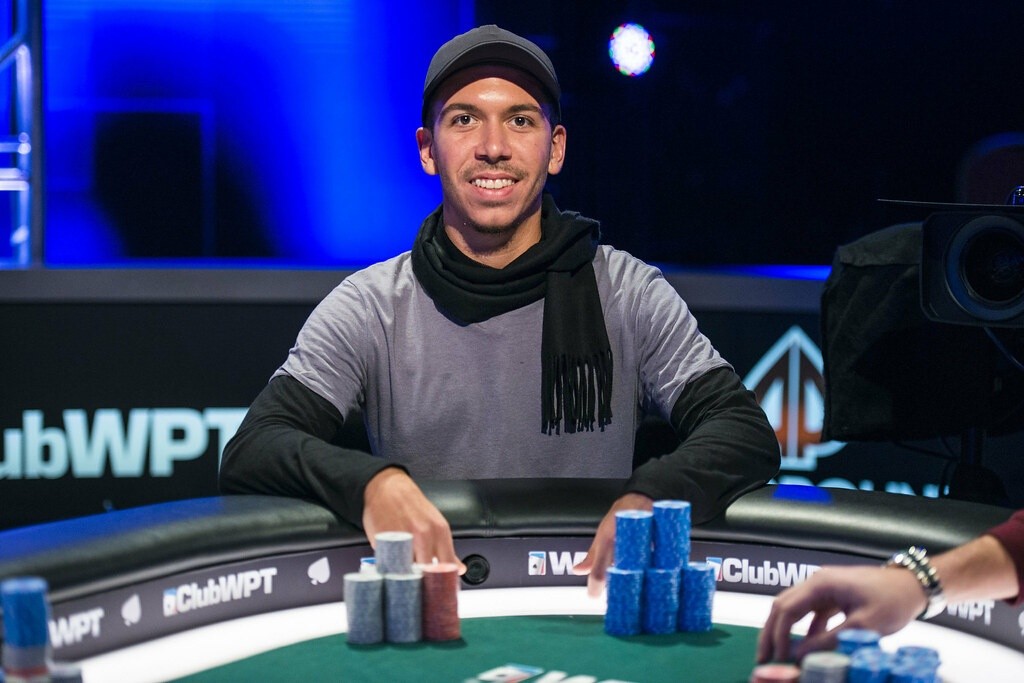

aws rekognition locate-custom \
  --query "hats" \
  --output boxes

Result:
[420,24,563,121]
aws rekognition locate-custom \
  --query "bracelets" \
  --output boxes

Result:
[889,547,946,620]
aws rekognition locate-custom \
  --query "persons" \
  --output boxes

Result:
[755,510,1023,662]
[819,138,1024,509]
[220,23,782,598]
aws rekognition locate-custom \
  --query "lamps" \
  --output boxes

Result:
[608,22,655,75]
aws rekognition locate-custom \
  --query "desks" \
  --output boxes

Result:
[0,478,1024,683]
